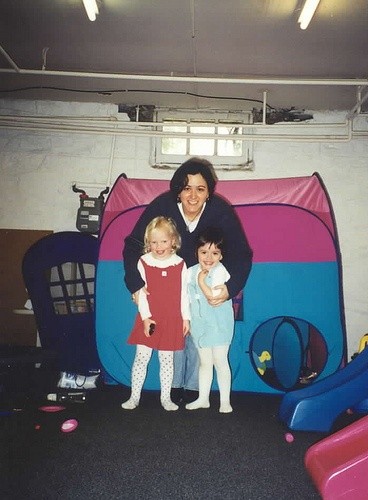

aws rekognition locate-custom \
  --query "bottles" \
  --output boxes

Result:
[47,391,87,403]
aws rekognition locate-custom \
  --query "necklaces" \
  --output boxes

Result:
[181,201,203,233]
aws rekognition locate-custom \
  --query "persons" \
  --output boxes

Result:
[122,162,253,413]
[186,232,235,413]
[121,216,191,411]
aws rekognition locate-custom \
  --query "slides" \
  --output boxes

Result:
[304,414,367,500]
[277,348,368,431]
